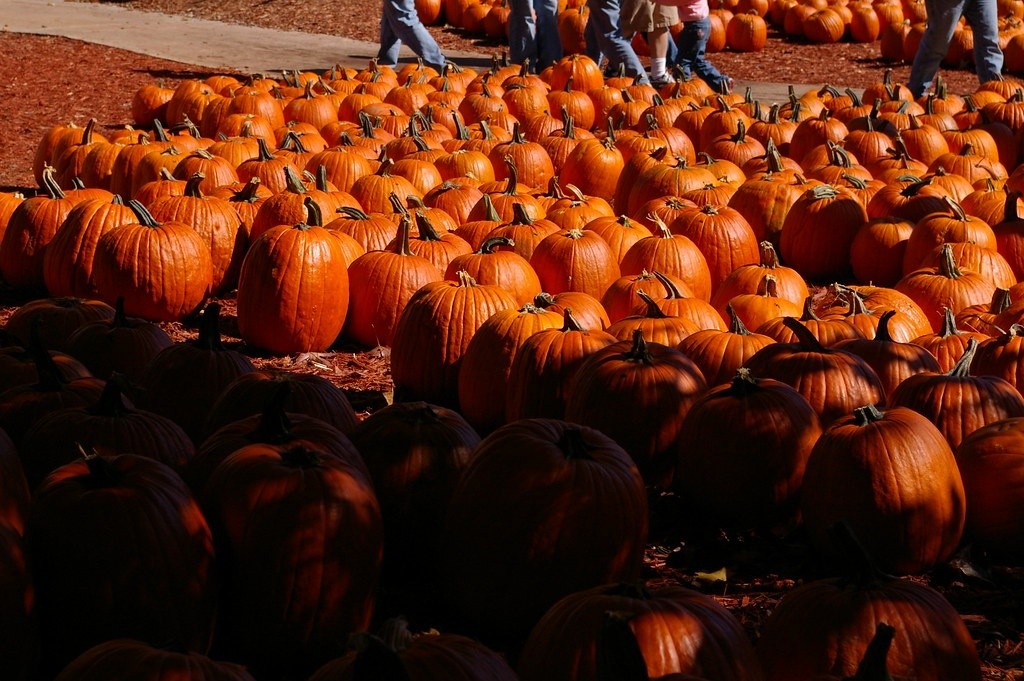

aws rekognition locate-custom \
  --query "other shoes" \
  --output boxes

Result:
[602,67,619,80]
[724,78,735,95]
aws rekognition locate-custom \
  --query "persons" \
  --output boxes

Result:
[583,0,735,95]
[906,0,1004,100]
[508,0,564,75]
[374,0,446,74]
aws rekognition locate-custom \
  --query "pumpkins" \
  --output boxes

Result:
[0,0,1024,681]
